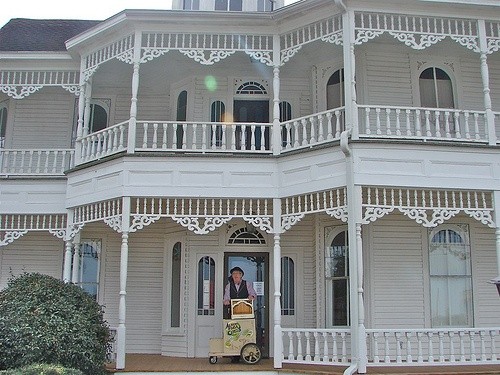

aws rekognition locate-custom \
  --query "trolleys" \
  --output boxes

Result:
[208,299,262,364]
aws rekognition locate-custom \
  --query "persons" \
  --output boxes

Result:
[223,266,258,317]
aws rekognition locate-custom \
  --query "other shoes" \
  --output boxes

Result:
[231,358,239,363]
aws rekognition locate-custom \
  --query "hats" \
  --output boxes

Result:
[230,267,244,276]
